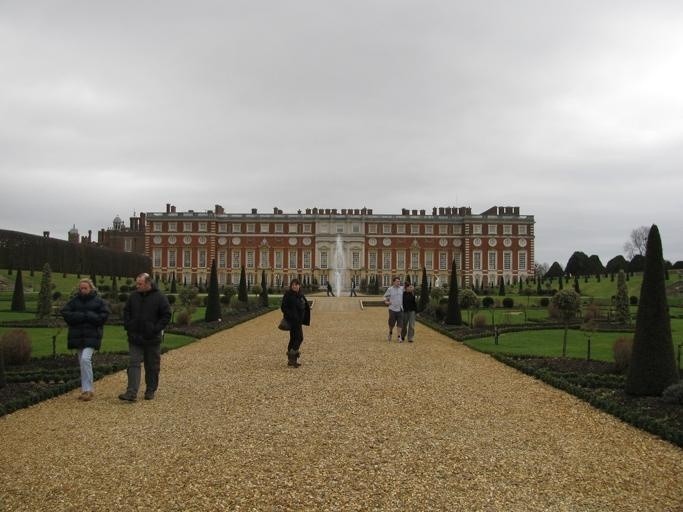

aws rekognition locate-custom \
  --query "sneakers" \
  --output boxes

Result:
[287,352,301,368]
[119,391,137,401]
[388,333,413,342]
[144,391,154,400]
[78,390,93,400]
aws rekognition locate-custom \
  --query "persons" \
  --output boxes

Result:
[281,279,310,367]
[326,281,335,296]
[60,278,109,400]
[383,277,416,344]
[349,278,357,297]
[119,273,172,402]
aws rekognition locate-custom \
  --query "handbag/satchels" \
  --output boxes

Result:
[303,295,310,326]
[278,318,290,330]
